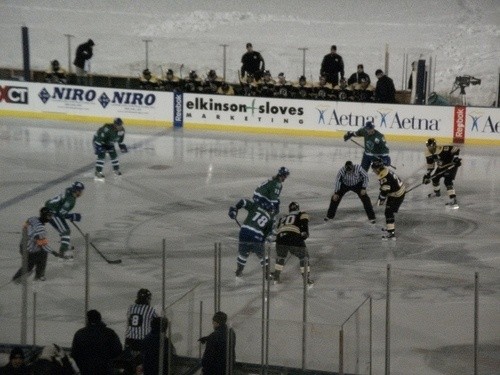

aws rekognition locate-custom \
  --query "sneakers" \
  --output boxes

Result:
[306,280,314,290]
[428,189,441,198]
[265,235,277,246]
[234,268,242,278]
[113,169,122,177]
[272,229,278,235]
[270,272,280,284]
[95,171,105,179]
[382,228,396,241]
[445,200,459,209]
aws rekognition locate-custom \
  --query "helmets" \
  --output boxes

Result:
[259,166,300,213]
[365,122,374,130]
[427,138,436,147]
[72,182,85,190]
[39,207,53,222]
[113,118,123,128]
[137,288,153,300]
[372,161,384,171]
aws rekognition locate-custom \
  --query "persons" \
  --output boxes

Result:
[12,207,53,282]
[348,64,371,89]
[215,81,234,95]
[241,42,265,75]
[73,37,95,78]
[374,69,396,103]
[139,69,182,93]
[323,161,377,224]
[409,61,428,91]
[270,201,315,285]
[320,45,345,87]
[93,117,127,179]
[183,70,223,94]
[0,342,75,375]
[240,70,293,86]
[422,138,463,205]
[43,59,67,85]
[314,76,374,91]
[70,309,122,375]
[198,312,235,375]
[292,75,313,88]
[228,197,276,281]
[253,166,290,227]
[124,288,176,375]
[371,161,406,238]
[44,181,85,252]
[343,122,389,175]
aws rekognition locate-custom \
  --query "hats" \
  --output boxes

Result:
[87,309,226,332]
[10,345,24,360]
[345,161,353,171]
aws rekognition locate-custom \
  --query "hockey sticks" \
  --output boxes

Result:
[101,149,131,153]
[235,218,241,228]
[350,138,404,170]
[405,164,455,193]
[71,221,122,264]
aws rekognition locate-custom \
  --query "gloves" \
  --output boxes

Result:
[453,157,461,166]
[229,206,237,218]
[344,131,356,142]
[377,194,386,205]
[70,213,82,222]
[423,173,436,184]
